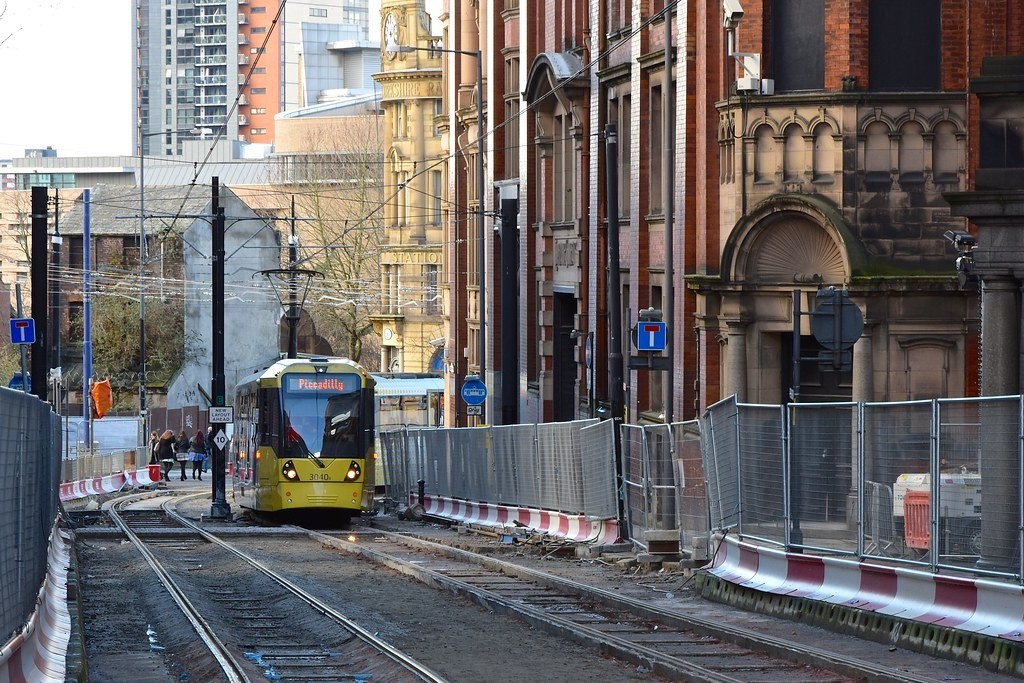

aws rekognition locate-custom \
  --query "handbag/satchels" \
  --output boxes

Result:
[154,442,160,452]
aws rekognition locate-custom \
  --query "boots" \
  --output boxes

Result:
[164,470,170,481]
[181,475,187,481]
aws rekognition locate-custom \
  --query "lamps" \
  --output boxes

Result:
[943,229,979,271]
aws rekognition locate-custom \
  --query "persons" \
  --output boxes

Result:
[148,427,212,481]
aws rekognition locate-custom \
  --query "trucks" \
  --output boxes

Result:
[891,469,983,557]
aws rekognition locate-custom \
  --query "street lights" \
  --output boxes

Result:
[139,127,202,469]
[384,44,486,429]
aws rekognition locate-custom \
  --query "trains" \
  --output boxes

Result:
[231,352,444,518]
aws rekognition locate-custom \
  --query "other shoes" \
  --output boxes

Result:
[193,475,195,479]
[160,472,164,480]
[198,476,202,481]
[202,470,207,474]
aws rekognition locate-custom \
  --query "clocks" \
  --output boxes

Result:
[382,12,407,61]
